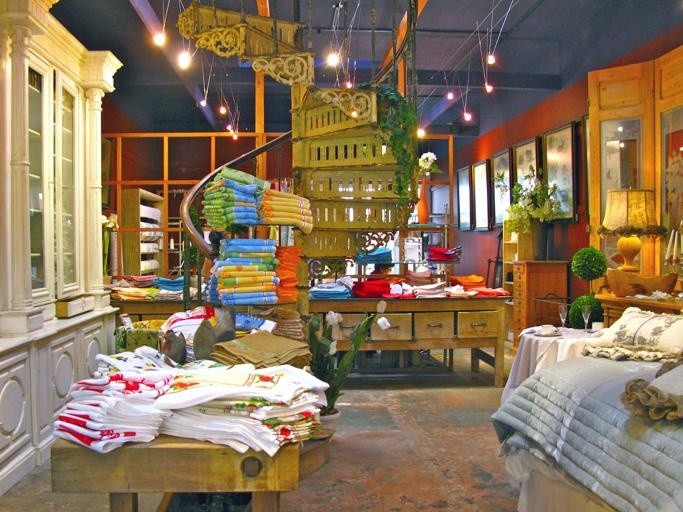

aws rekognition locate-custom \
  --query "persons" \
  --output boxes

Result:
[367,263,395,280]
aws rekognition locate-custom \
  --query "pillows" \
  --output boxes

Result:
[581,306,683,362]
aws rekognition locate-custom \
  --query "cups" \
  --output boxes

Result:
[542,325,553,336]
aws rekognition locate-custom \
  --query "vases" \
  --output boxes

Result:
[418,175,428,224]
[546,223,556,262]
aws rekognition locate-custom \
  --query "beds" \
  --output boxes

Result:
[490,356,683,512]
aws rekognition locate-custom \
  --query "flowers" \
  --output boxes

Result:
[418,152,442,176]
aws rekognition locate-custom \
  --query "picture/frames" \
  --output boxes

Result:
[455,120,579,233]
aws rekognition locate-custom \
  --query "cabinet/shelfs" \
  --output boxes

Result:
[511,261,568,357]
[122,187,164,275]
[0,0,124,336]
[103,132,454,280]
[593,294,683,329]
[157,190,224,279]
[502,219,544,343]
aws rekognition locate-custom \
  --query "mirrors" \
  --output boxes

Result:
[599,116,642,269]
[661,104,683,280]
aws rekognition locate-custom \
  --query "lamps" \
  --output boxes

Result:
[417,0,513,139]
[597,186,667,271]
[326,3,359,117]
[153,0,239,141]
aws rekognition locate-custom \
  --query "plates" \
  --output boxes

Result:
[535,332,562,337]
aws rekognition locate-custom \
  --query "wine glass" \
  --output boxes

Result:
[558,304,567,334]
[580,305,591,337]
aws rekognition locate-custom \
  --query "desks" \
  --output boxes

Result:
[109,296,507,388]
[500,327,602,405]
[51,437,301,512]
